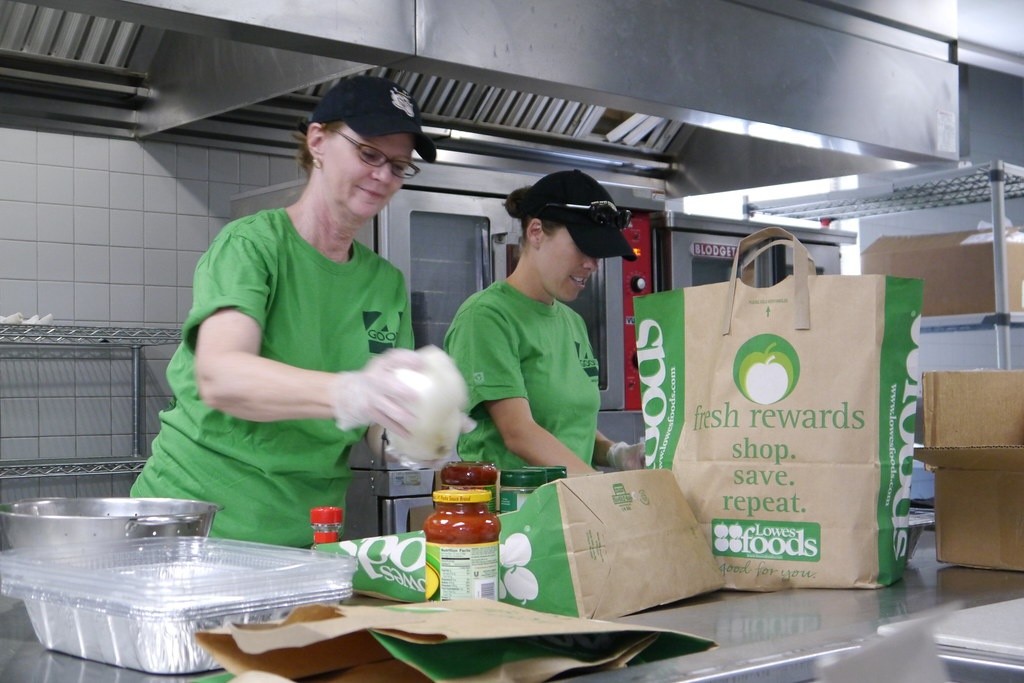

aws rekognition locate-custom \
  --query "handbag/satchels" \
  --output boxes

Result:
[633,227,924,590]
[193,597,719,683]
[314,468,724,624]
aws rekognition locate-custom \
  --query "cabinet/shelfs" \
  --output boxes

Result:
[744,158,1024,370]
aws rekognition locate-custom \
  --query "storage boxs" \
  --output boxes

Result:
[0,535,359,675]
[916,371,1024,574]
[861,226,1023,318]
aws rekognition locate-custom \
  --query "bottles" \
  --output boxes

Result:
[312,506,343,542]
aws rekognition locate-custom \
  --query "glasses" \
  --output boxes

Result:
[325,127,421,179]
[535,201,632,229]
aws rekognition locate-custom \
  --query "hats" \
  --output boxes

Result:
[524,170,637,261]
[307,75,437,163]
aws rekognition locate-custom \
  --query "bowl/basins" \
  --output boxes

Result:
[0,497,225,551]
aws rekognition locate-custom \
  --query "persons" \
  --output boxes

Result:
[131,74,435,547]
[440,169,650,481]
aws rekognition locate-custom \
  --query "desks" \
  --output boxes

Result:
[1,546,1024,683]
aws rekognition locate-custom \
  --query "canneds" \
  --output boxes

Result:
[423,489,503,603]
[440,461,567,514]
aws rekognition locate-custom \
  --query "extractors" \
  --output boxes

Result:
[0,0,960,187]
